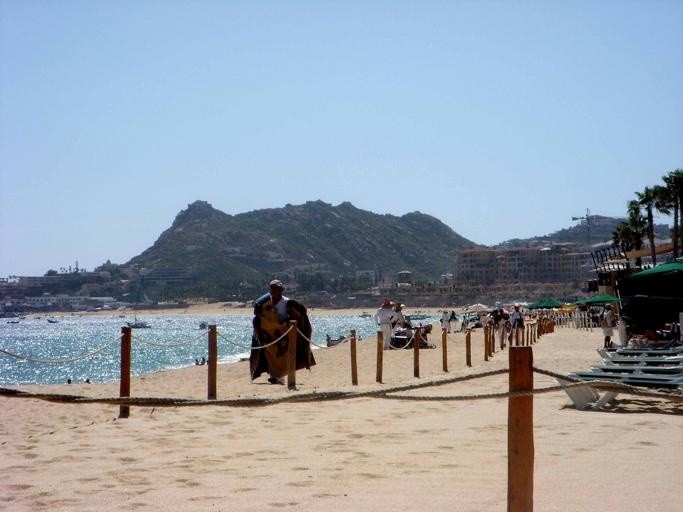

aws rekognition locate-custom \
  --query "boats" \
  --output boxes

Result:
[19,316,25,319]
[6,321,18,323]
[326,337,346,346]
[47,319,58,323]
[34,316,40,320]
[127,321,151,329]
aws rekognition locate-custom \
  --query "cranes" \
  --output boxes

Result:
[571,208,613,243]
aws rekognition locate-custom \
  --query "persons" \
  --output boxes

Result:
[439,304,526,333]
[253,279,292,384]
[604,303,615,348]
[374,299,432,350]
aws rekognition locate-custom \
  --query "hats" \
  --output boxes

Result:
[267,280,287,288]
[380,300,395,308]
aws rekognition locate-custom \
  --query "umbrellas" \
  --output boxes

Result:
[528,293,623,320]
[630,262,683,285]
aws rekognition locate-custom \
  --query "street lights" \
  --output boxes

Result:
[198,322,208,329]
[408,313,431,320]
[359,312,371,318]
